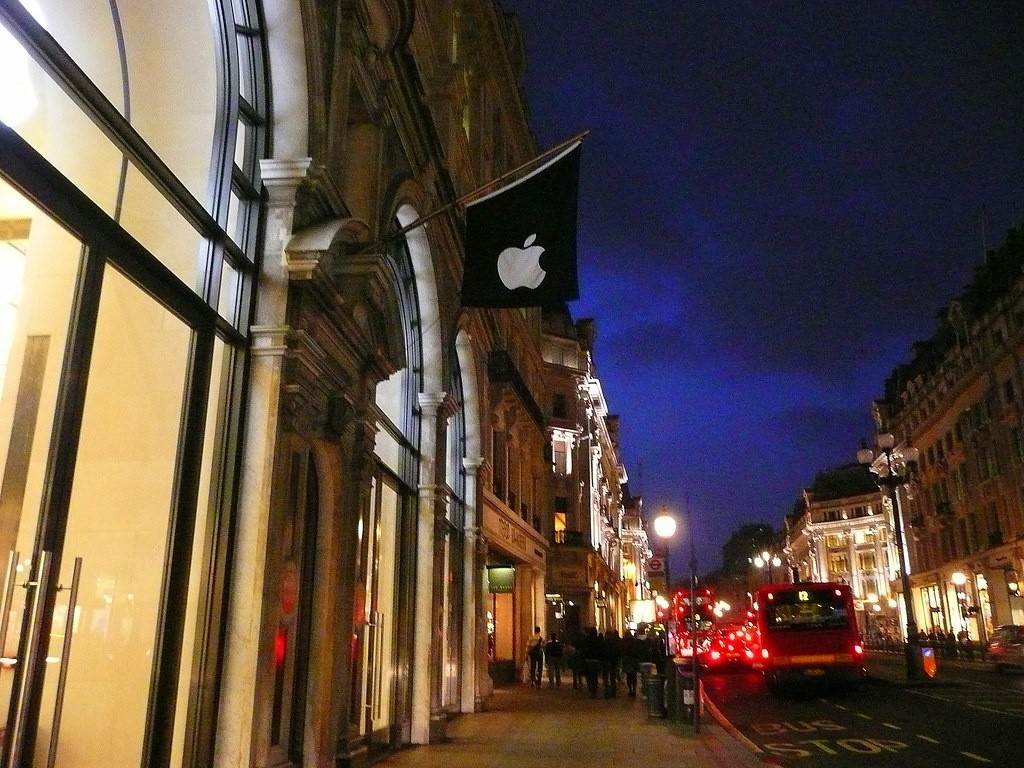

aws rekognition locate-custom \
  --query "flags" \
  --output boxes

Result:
[459,133,587,306]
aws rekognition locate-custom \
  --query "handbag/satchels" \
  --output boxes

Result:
[528,638,542,659]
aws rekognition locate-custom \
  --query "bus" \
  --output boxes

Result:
[742,583,867,698]
[716,622,742,664]
[675,589,721,667]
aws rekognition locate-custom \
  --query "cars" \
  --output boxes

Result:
[989,624,1024,672]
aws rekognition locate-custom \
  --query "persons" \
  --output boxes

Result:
[859,626,969,658]
[545,632,563,688]
[525,626,545,689]
[569,619,666,699]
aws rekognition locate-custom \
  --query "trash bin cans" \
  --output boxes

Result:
[671,658,701,725]
[643,675,668,717]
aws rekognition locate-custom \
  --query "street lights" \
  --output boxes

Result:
[856,427,925,678]
